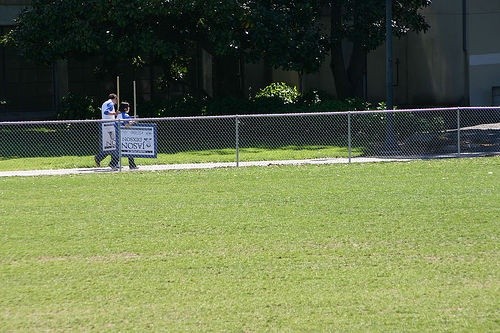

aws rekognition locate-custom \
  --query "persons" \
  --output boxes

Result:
[95,93,123,168]
[107,101,140,171]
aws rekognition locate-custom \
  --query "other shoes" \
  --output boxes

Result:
[94,156,101,167]
[116,164,123,168]
[109,164,118,171]
[131,166,139,169]
